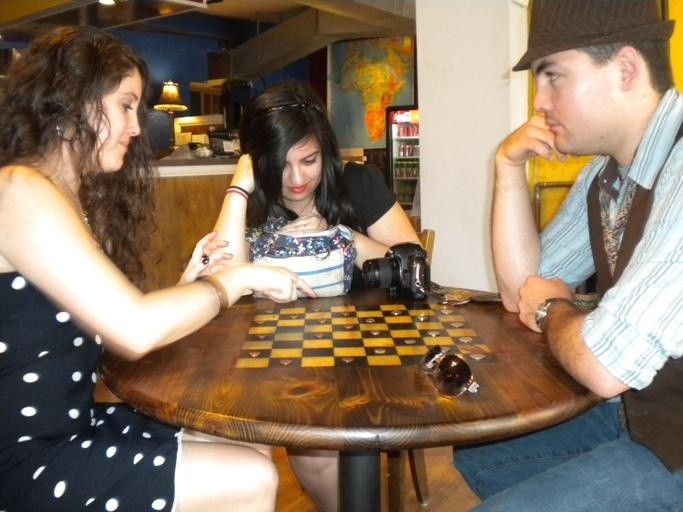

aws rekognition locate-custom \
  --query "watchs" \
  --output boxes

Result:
[534,296,578,335]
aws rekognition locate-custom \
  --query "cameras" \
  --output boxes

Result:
[362,243,430,301]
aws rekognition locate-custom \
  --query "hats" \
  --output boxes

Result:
[509,0,677,73]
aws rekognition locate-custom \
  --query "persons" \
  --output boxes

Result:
[0,25,319,512]
[142,78,171,159]
[202,78,429,512]
[449,0,682,512]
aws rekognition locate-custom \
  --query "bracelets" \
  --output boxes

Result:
[224,185,251,200]
[193,274,229,321]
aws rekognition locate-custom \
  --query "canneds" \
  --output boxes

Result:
[398,126,419,156]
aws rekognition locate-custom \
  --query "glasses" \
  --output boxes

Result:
[418,340,480,400]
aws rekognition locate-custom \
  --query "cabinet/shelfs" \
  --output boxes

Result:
[386,105,419,210]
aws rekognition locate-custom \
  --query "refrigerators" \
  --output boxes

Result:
[382,105,418,209]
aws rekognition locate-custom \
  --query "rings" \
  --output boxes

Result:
[303,223,307,231]
[201,253,210,266]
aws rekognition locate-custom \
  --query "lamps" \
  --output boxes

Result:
[153,81,188,151]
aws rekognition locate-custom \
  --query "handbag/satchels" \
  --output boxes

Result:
[245,214,357,300]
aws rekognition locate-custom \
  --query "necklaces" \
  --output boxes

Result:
[31,149,106,252]
[294,188,316,218]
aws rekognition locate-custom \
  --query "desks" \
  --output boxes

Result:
[97,281,620,509]
